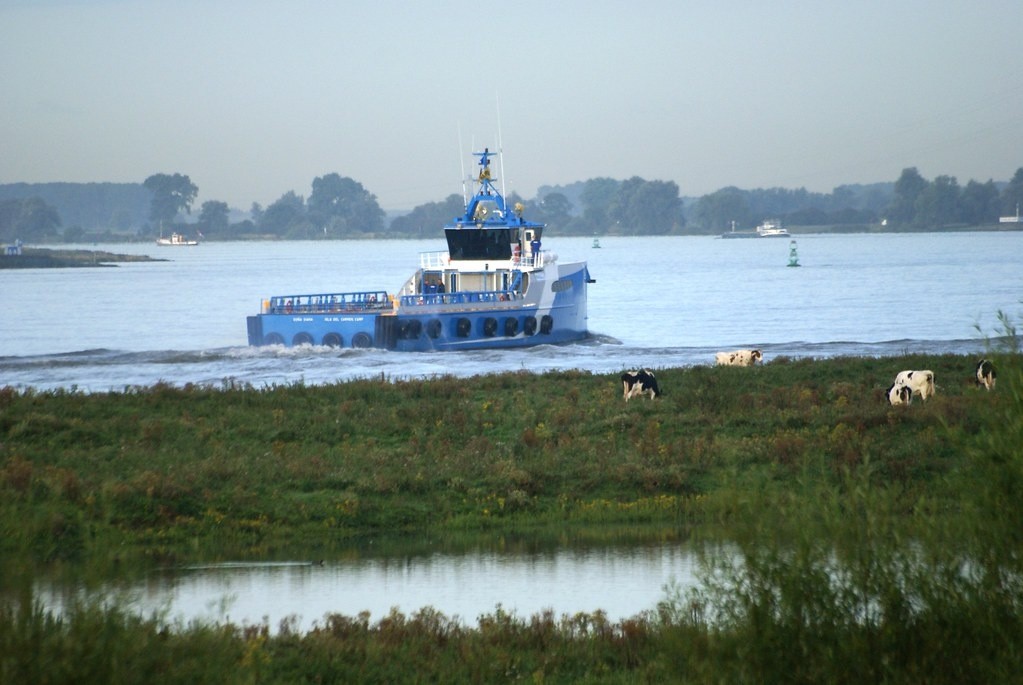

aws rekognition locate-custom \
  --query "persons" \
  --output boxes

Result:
[422,280,430,305]
[530,235,541,266]
[437,279,445,303]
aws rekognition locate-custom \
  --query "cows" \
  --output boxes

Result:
[976,359,997,390]
[621,370,660,403]
[716,349,764,367]
[885,369,935,406]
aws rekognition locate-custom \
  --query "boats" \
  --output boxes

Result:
[756,222,791,239]
[156,232,198,247]
[247,90,597,352]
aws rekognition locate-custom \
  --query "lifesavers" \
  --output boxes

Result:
[286,300,293,313]
[505,317,519,336]
[457,317,472,337]
[525,316,537,335]
[351,332,372,349]
[429,319,442,339]
[397,318,410,339]
[408,319,423,339]
[264,332,284,347]
[291,331,314,346]
[541,315,553,335]
[322,332,343,348]
[484,317,498,336]
